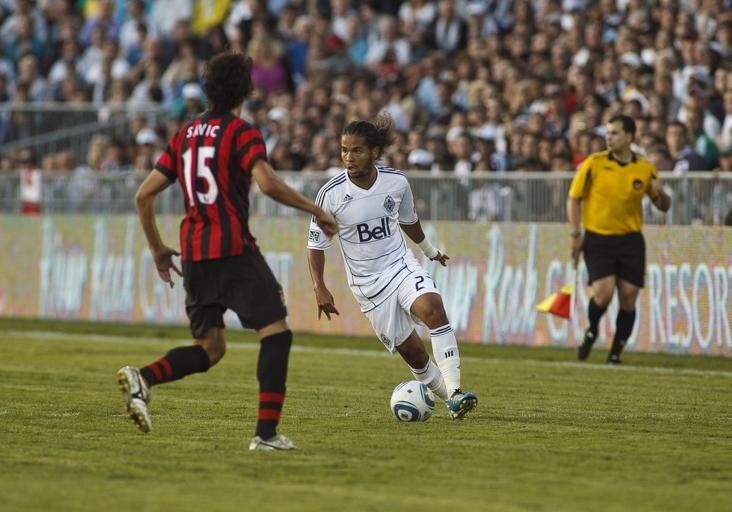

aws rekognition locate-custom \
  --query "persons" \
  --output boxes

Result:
[2,1,732,227]
[307,110,478,421]
[564,113,673,365]
[115,50,340,456]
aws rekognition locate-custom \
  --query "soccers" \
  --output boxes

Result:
[389,379,435,422]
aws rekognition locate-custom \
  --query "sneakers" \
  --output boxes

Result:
[579,328,597,359]
[116,366,153,433]
[444,388,478,421]
[606,355,620,365]
[249,434,302,452]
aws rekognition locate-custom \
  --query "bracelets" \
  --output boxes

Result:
[569,230,582,239]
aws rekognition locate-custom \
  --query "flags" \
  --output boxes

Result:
[535,284,571,318]
